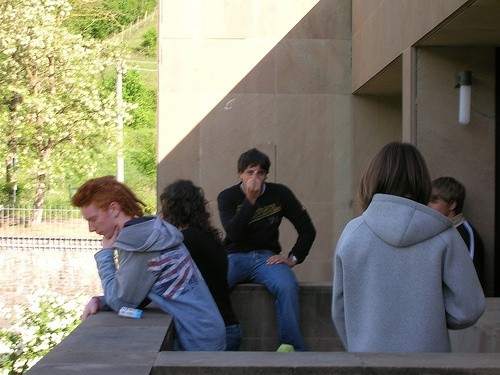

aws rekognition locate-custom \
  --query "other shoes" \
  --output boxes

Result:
[277,344,295,353]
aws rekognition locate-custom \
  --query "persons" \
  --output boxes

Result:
[71,175,228,351]
[428,176,478,273]
[159,179,242,331]
[331,141,486,353]
[217,149,318,351]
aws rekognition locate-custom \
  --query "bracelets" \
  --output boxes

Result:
[290,254,298,263]
